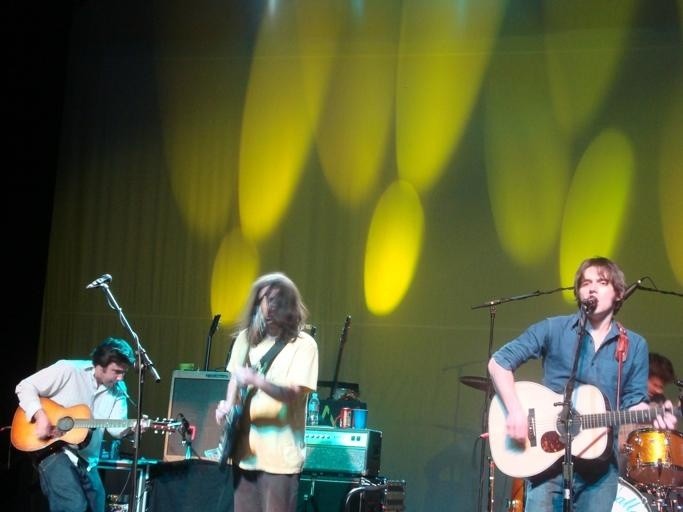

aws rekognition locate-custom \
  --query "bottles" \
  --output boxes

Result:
[307,393,321,425]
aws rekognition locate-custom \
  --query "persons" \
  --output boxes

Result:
[483,255,679,511]
[608,349,675,454]
[213,270,319,512]
[14,337,152,512]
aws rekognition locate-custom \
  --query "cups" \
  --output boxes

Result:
[353,408,368,430]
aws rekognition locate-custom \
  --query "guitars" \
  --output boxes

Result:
[10,396,183,452]
[488,380,683,479]
[218,367,258,472]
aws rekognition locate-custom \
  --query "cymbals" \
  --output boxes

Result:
[459,376,492,390]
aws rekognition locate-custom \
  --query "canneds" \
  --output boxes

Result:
[340,408,351,428]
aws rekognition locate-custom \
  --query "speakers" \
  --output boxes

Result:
[162,371,230,466]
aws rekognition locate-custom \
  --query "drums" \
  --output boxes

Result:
[611,477,683,512]
[624,428,683,487]
[505,478,525,512]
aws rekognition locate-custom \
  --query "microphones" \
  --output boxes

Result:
[84,269,114,293]
[581,293,597,313]
[177,410,190,430]
[613,277,640,316]
